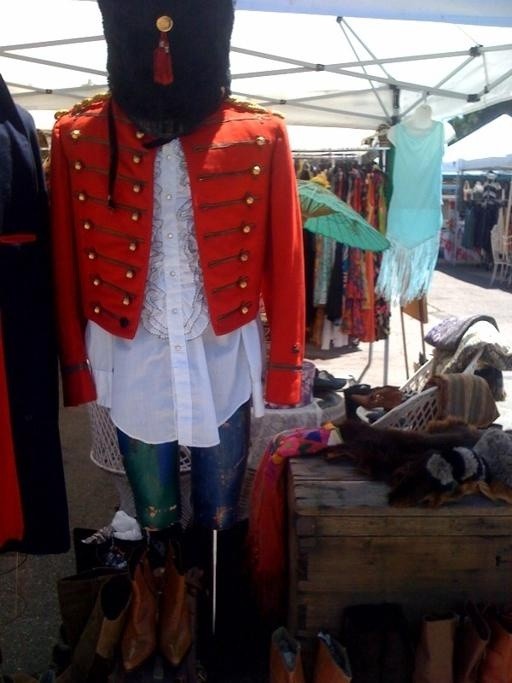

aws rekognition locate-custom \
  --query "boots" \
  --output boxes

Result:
[412,612,457,683]
[48,526,134,683]
[268,626,305,683]
[314,632,354,683]
[457,614,511,682]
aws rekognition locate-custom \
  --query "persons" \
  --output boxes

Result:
[48,0,311,556]
[370,103,457,314]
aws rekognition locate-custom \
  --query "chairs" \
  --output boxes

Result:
[364,331,488,431]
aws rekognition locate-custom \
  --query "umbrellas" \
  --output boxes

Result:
[289,164,391,271]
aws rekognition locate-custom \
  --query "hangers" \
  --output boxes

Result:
[290,146,388,177]
[385,90,457,145]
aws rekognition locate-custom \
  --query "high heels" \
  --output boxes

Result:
[158,537,198,667]
[121,552,158,674]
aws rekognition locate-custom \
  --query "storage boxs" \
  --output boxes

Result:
[285,458,511,640]
